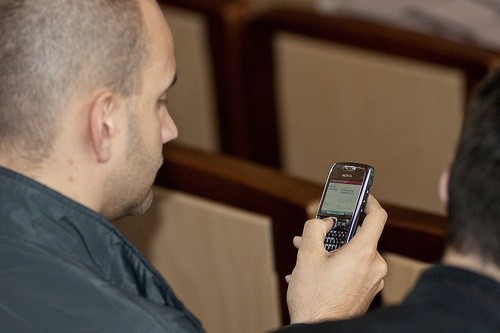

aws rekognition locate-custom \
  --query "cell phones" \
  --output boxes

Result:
[313,162,375,252]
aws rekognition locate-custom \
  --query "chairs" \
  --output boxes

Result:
[152,0,235,156]
[111,141,323,333]
[240,7,480,231]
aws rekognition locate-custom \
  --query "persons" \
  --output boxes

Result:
[0,0,391,333]
[262,60,499,333]
